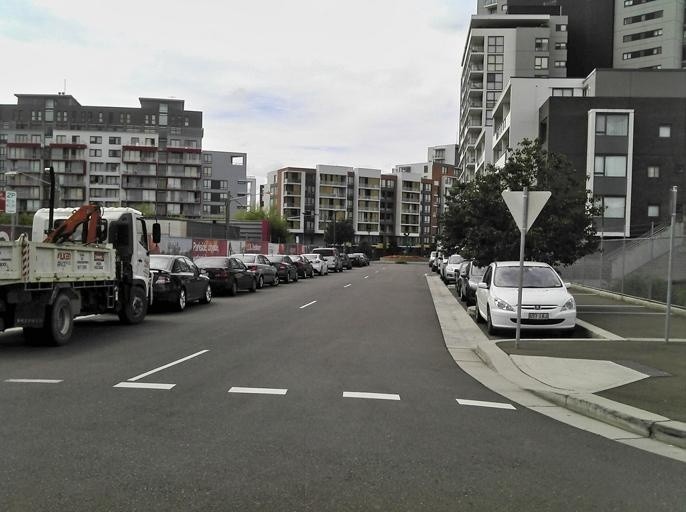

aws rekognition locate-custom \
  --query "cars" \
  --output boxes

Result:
[264,248,369,282]
[429,252,488,303]
[477,262,576,333]
[230,254,278,288]
[148,254,210,312]
[194,255,257,296]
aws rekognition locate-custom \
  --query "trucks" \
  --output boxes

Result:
[1,205,159,346]
